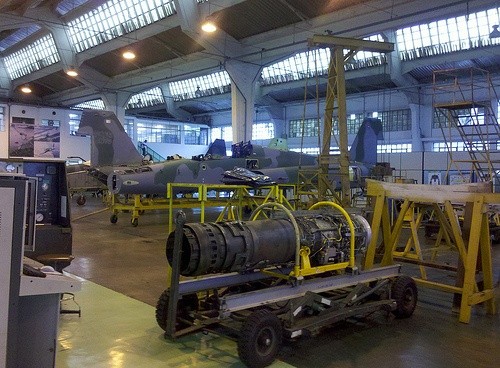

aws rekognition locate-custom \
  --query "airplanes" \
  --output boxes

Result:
[105,117,396,193]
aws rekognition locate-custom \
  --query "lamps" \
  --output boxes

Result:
[22,84,32,93]
[123,29,135,59]
[67,65,78,77]
[202,2,216,33]
